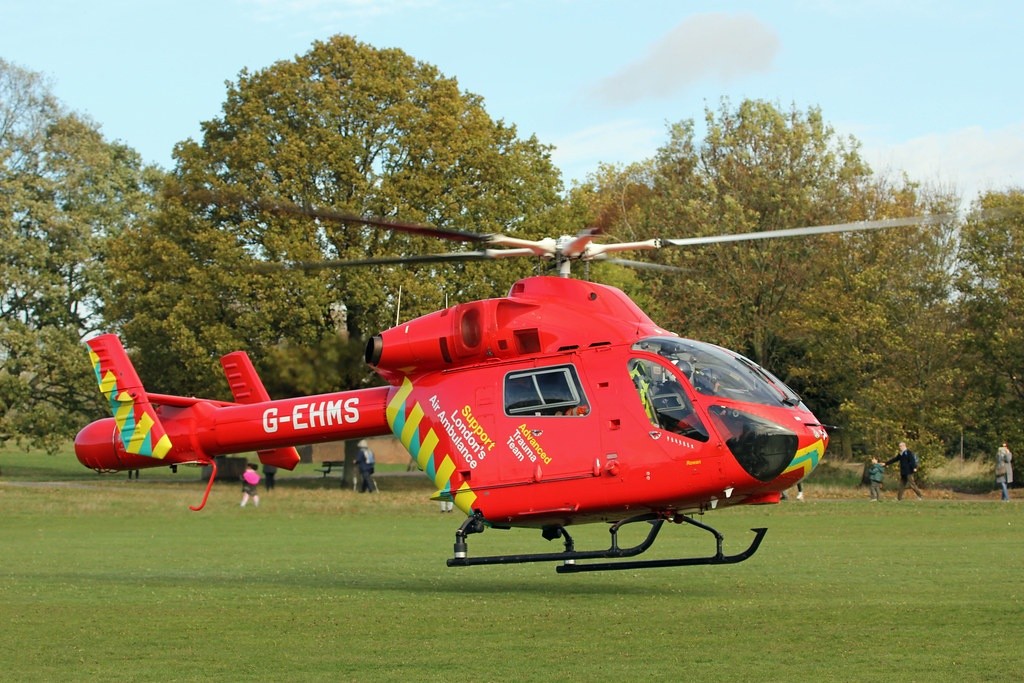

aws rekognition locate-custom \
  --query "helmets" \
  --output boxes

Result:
[665,359,691,382]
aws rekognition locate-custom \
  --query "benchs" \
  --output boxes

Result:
[315,461,345,477]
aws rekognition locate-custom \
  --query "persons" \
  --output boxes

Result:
[868,455,884,503]
[354,440,375,494]
[796,482,803,501]
[996,442,1013,503]
[653,360,744,447]
[566,394,591,417]
[240,463,262,512]
[262,465,277,489]
[879,442,926,502]
[128,470,139,482]
[169,464,178,474]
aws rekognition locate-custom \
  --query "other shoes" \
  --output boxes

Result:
[780,495,788,500]
[797,492,804,500]
[870,498,877,502]
[448,510,452,513]
[442,509,446,512]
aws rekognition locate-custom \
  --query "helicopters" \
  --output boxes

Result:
[72,189,1018,573]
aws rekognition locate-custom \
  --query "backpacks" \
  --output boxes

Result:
[905,448,918,467]
[360,449,374,464]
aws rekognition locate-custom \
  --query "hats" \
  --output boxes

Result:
[357,439,368,448]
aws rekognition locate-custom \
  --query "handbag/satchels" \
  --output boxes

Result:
[995,466,1006,477]
